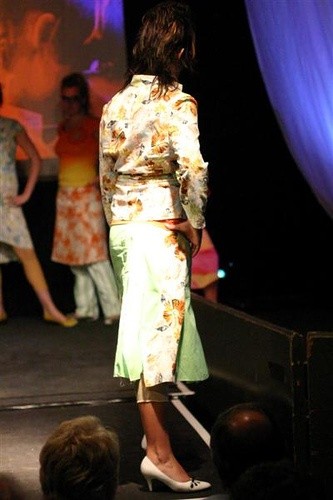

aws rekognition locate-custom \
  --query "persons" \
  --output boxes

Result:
[99,6,212,493]
[0,0,122,158]
[0,88,77,328]
[40,403,323,500]
[52,72,124,325]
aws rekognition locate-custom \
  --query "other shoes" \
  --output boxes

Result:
[43,309,79,328]
[0,309,7,321]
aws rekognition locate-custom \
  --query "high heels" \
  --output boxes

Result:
[141,434,151,452]
[140,455,211,493]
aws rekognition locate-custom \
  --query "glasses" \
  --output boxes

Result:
[62,95,80,104]
